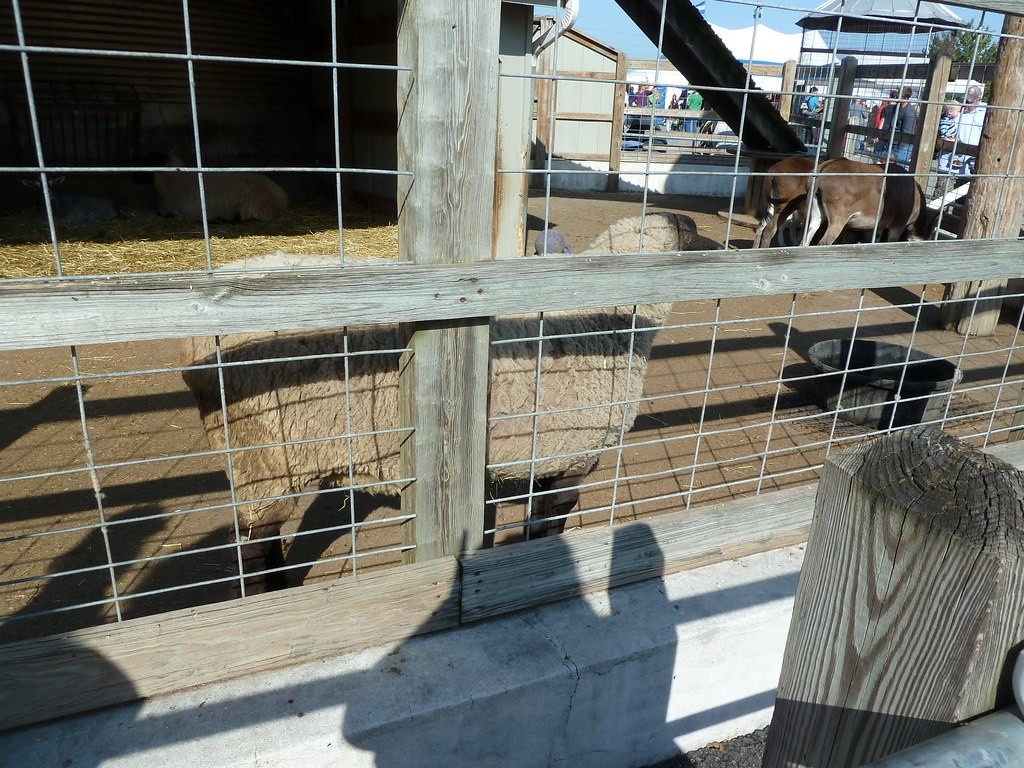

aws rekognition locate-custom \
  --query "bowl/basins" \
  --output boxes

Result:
[808,338,964,428]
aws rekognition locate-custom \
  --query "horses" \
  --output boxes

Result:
[744,154,947,250]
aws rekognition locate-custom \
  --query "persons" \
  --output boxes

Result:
[625,84,714,143]
[797,86,990,186]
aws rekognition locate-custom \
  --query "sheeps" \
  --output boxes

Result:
[182,210,728,603]
